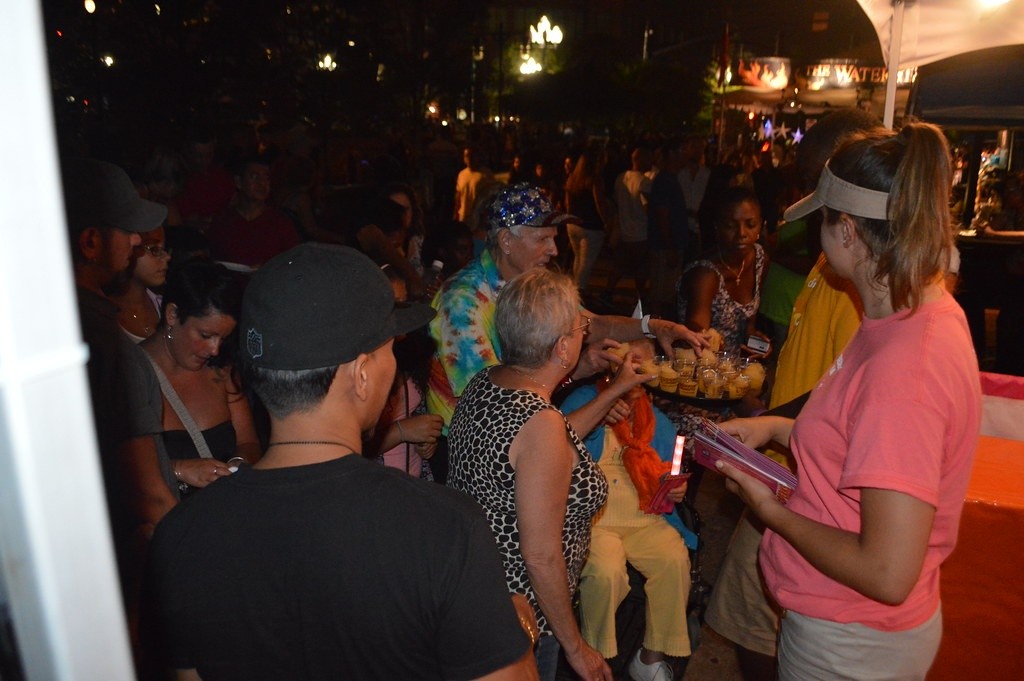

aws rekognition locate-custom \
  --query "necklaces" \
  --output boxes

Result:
[268,441,359,455]
[515,369,551,398]
[127,308,151,337]
[717,245,745,287]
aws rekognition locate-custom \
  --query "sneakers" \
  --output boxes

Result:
[629,648,673,681]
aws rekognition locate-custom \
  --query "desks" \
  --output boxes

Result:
[955,230,1024,374]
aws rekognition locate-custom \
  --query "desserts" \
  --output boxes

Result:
[605,327,768,399]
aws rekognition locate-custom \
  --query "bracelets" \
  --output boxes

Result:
[560,375,573,388]
[397,419,404,442]
[518,615,537,644]
[179,458,188,488]
[641,314,656,338]
[226,457,243,463]
[174,459,184,490]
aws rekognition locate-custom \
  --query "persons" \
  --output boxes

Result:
[702,108,885,657]
[445,267,665,681]
[137,244,538,681]
[687,123,982,681]
[61,107,812,550]
[421,182,706,481]
[950,134,1024,375]
[556,336,699,681]
[356,240,444,478]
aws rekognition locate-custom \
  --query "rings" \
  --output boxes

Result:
[214,467,216,474]
[431,445,434,449]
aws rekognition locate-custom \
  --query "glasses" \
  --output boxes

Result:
[140,244,171,256]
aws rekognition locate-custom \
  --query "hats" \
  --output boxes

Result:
[64,160,168,233]
[783,157,892,223]
[238,240,438,370]
[489,181,582,228]
